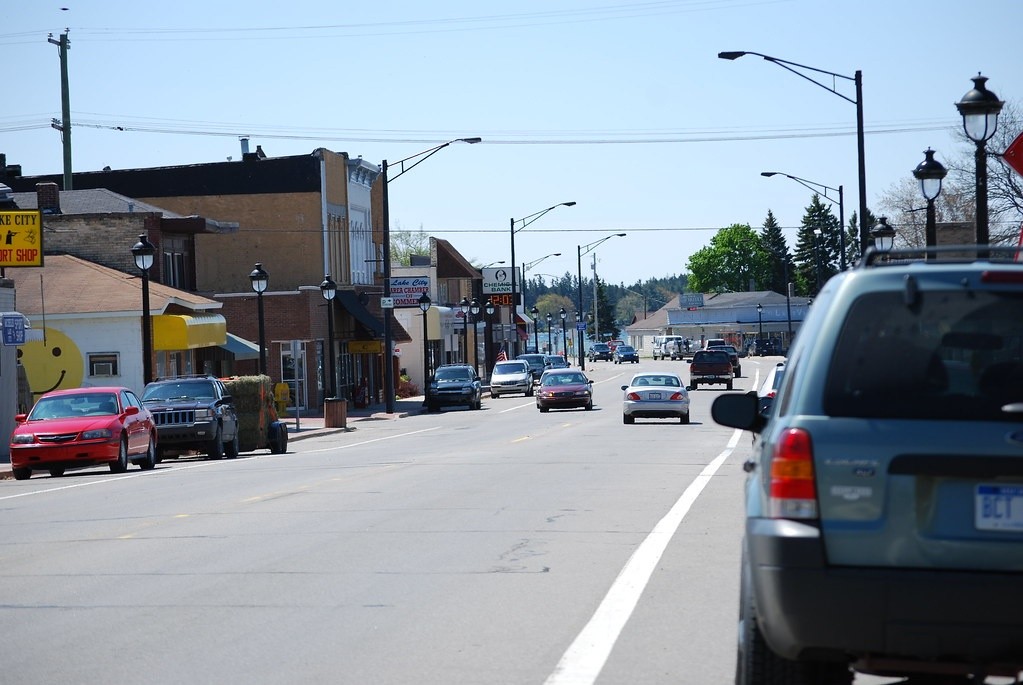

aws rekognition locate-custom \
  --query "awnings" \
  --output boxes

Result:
[152,311,227,351]
[518,328,528,341]
[516,313,534,324]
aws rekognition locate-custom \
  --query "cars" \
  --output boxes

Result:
[748,339,774,356]
[620,372,692,424]
[536,369,594,413]
[487,359,536,398]
[613,345,640,364]
[515,354,572,380]
[9,386,158,480]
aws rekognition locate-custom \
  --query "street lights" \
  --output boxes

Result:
[559,307,567,364]
[523,253,561,352]
[460,296,495,377]
[511,202,577,357]
[382,136,482,414]
[760,171,845,272]
[718,51,869,259]
[248,261,271,379]
[575,311,581,366]
[578,233,626,370]
[912,146,947,259]
[319,276,338,398]
[531,305,540,355]
[129,232,157,387]
[954,71,1006,258]
[418,291,431,404]
[546,312,553,355]
[757,303,763,357]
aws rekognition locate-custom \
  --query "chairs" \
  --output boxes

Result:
[639,379,649,384]
[100,402,117,412]
[665,379,673,385]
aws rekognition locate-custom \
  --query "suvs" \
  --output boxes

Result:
[589,340,625,362]
[687,339,741,390]
[710,245,1023,685]
[139,373,239,463]
[651,335,685,360]
[434,362,482,410]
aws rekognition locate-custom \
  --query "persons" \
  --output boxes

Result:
[571,374,582,381]
[566,338,572,356]
[747,341,757,358]
[550,377,561,385]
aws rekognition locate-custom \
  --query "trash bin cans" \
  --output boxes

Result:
[323,397,347,430]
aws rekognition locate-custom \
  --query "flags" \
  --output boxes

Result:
[496,346,507,360]
